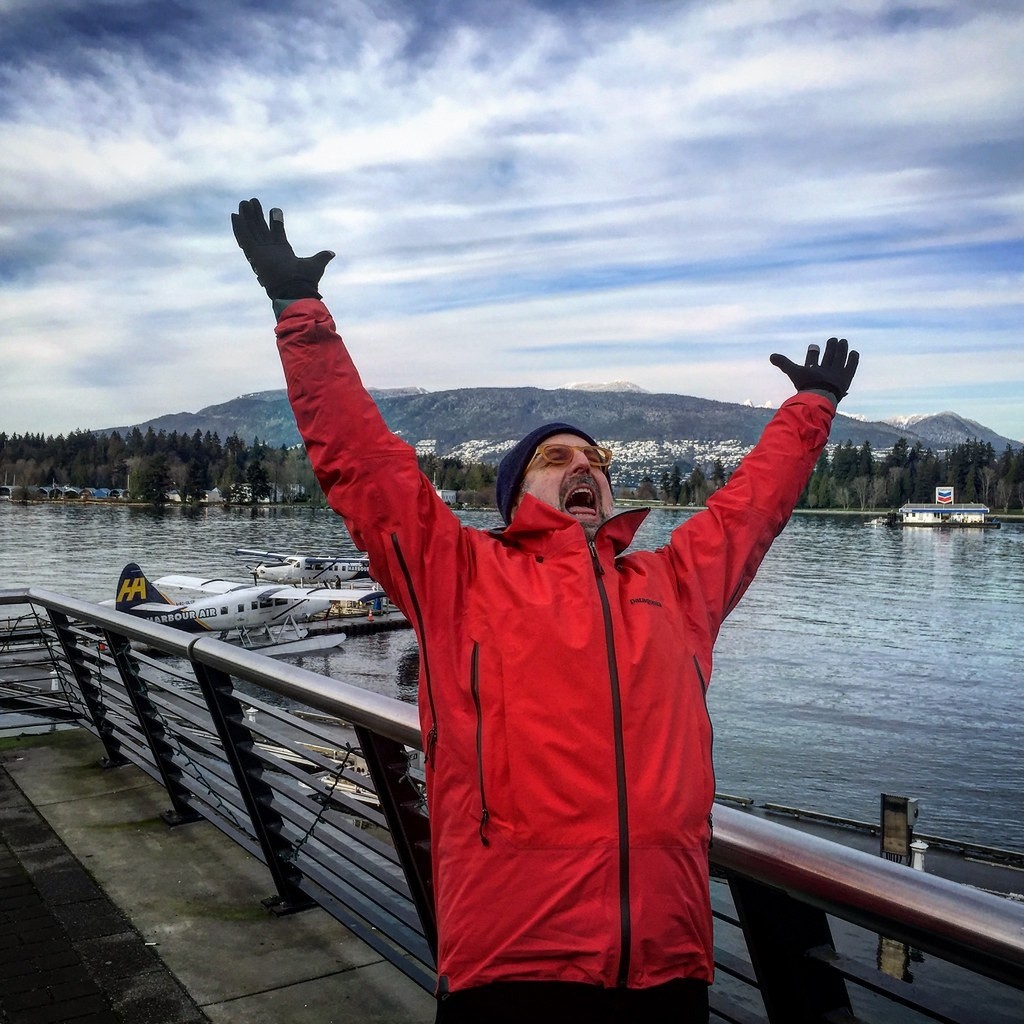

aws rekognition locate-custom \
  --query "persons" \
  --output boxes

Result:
[230,197,858,1024]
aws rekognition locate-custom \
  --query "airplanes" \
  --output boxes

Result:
[98,546,390,657]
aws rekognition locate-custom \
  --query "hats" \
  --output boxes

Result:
[496,423,598,526]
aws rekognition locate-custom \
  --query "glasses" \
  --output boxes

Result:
[523,446,612,475]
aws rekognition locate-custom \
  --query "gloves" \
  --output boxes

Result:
[231,198,336,300]
[770,337,860,406]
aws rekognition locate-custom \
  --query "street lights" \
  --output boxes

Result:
[367,610,374,621]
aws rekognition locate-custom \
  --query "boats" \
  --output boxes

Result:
[864,486,1001,529]
